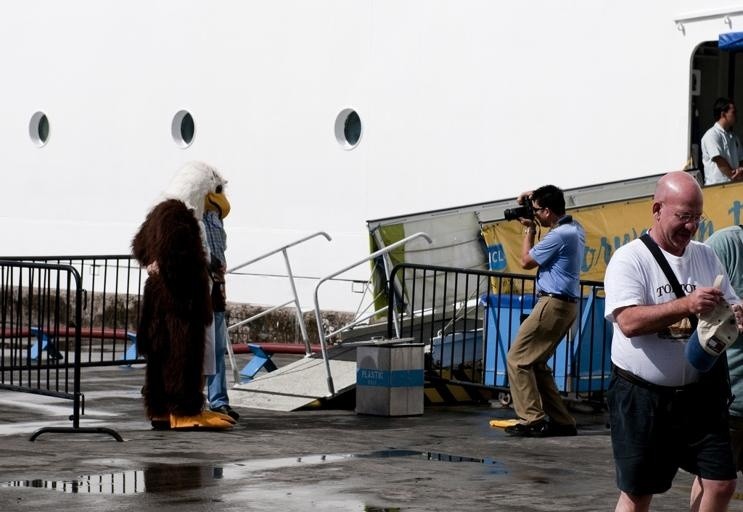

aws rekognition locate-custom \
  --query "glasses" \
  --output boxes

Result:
[662,202,705,224]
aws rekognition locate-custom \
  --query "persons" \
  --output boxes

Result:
[604,171,742,510]
[200,210,238,423]
[702,224,743,471]
[700,98,743,186]
[504,186,586,438]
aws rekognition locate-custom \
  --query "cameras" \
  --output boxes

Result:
[503,194,537,222]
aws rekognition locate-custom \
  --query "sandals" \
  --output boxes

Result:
[505,419,544,437]
[544,424,577,436]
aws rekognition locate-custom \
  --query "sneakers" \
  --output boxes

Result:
[209,405,234,416]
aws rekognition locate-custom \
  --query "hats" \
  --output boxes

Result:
[684,275,739,373]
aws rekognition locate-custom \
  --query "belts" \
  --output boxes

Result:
[537,291,579,303]
[614,367,684,392]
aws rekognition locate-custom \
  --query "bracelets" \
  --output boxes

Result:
[525,229,537,234]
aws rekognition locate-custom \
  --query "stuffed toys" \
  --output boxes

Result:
[131,165,236,430]
